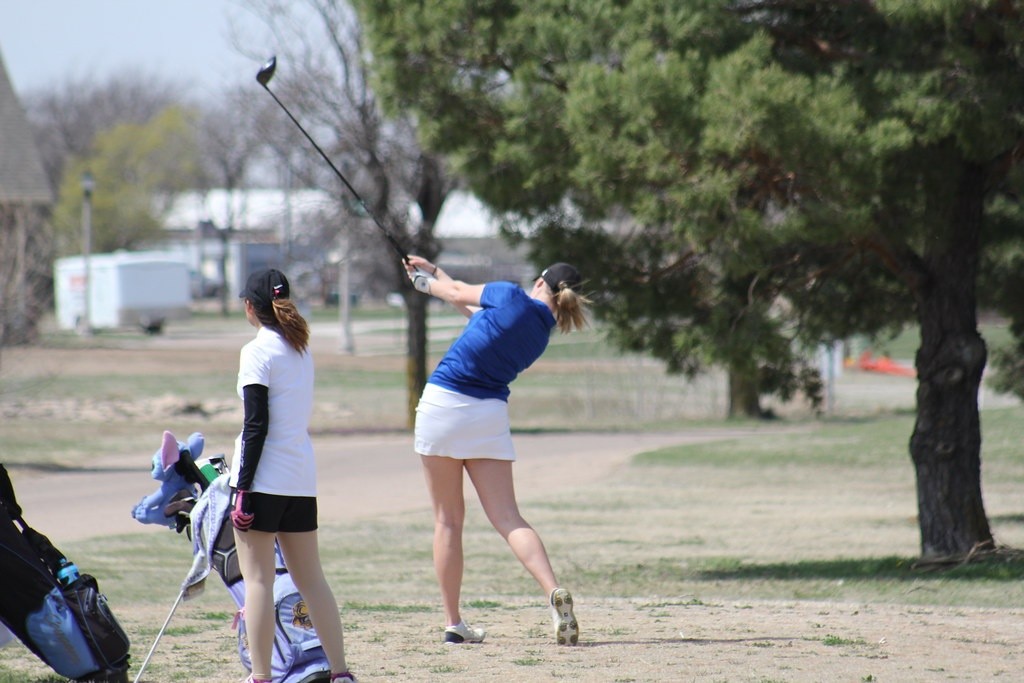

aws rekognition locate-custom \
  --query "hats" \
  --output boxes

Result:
[239,268,289,303]
[531,262,581,295]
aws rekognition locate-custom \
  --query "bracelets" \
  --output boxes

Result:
[432,264,438,276]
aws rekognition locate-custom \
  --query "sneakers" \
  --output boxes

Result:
[550,588,579,646]
[445,620,488,645]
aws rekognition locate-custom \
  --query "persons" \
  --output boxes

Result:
[403,251,600,648]
[229,266,355,683]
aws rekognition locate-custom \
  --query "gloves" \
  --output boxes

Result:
[230,490,255,532]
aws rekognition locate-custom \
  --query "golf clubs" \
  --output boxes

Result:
[163,453,229,541]
[257,54,420,275]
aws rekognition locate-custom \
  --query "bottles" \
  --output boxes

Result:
[56,558,79,587]
